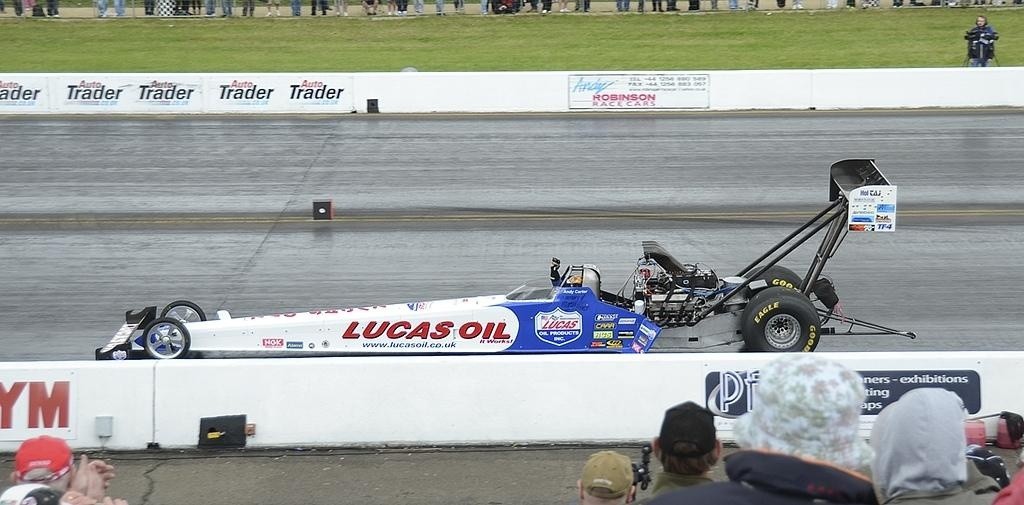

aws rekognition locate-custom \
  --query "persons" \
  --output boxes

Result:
[868,385,1007,504]
[7,434,78,500]
[963,15,999,66]
[650,400,721,496]
[575,450,636,505]
[1,452,132,505]
[632,349,886,505]
[0,0,1019,21]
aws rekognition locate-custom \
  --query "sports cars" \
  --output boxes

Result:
[94,158,918,361]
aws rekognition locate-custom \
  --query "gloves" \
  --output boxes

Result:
[551,256,560,271]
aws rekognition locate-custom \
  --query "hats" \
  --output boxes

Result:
[732,356,877,472]
[580,449,635,499]
[659,401,716,457]
[14,435,75,485]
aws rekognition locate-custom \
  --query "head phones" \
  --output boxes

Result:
[975,21,988,26]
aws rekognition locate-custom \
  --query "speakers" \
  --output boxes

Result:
[312,198,333,220]
[367,98,378,114]
[197,412,247,449]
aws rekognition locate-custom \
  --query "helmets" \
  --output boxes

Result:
[566,275,583,284]
